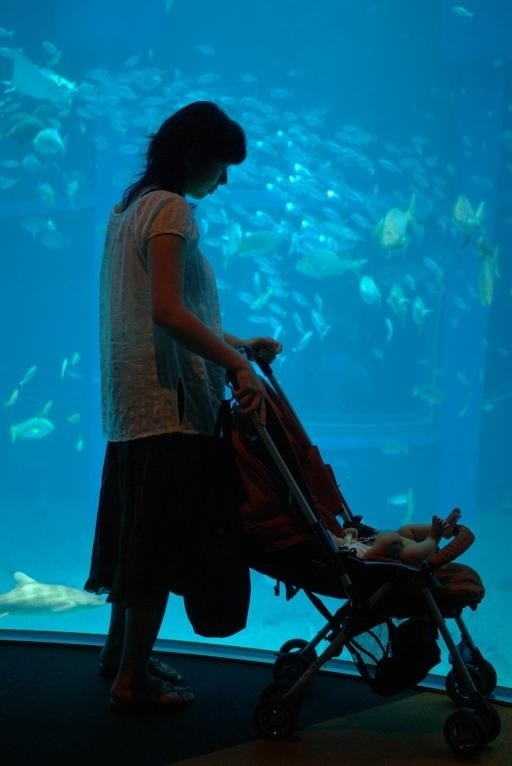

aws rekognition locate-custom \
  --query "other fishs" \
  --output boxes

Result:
[0,41,512,455]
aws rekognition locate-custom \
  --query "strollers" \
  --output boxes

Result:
[216,341,502,757]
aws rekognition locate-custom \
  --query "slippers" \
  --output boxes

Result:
[108,672,195,711]
[96,648,183,687]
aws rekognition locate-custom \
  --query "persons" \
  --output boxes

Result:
[83,100,282,710]
[326,507,462,564]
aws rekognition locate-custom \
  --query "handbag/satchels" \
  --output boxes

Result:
[183,397,253,638]
